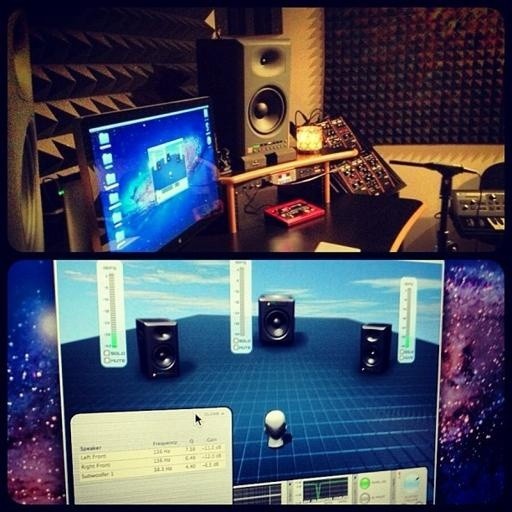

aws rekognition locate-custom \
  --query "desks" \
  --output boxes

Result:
[155,188,427,257]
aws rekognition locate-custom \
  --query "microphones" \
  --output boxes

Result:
[389,159,476,177]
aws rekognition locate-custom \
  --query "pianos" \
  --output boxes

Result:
[450,189,506,242]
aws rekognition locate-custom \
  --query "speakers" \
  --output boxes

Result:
[258,294,295,348]
[359,322,392,378]
[136,317,180,380]
[156,154,180,171]
[198,37,298,171]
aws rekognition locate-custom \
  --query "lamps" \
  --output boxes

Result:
[293,105,329,152]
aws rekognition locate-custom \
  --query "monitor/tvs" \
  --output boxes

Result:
[77,95,227,254]
